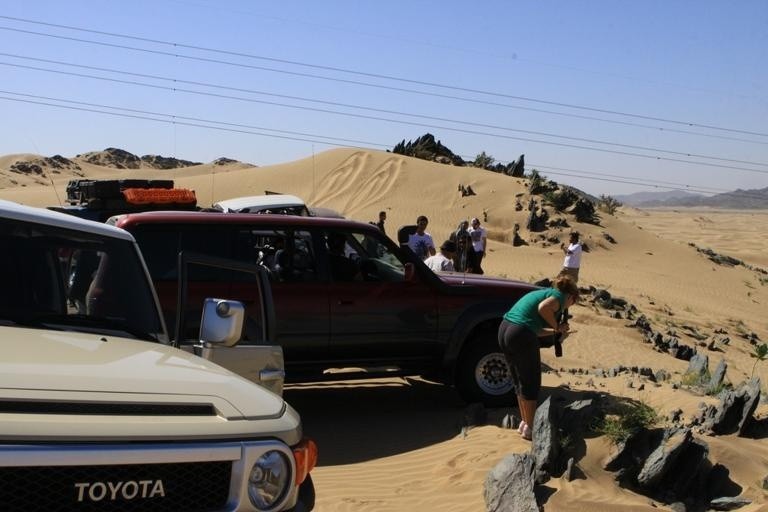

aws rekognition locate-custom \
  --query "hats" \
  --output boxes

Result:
[471,217,479,227]
[569,230,580,237]
[438,239,458,252]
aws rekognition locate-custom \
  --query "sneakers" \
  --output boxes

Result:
[522,423,533,441]
[517,420,526,434]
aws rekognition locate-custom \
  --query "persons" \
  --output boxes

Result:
[557,232,582,282]
[499,277,577,439]
[359,211,487,275]
